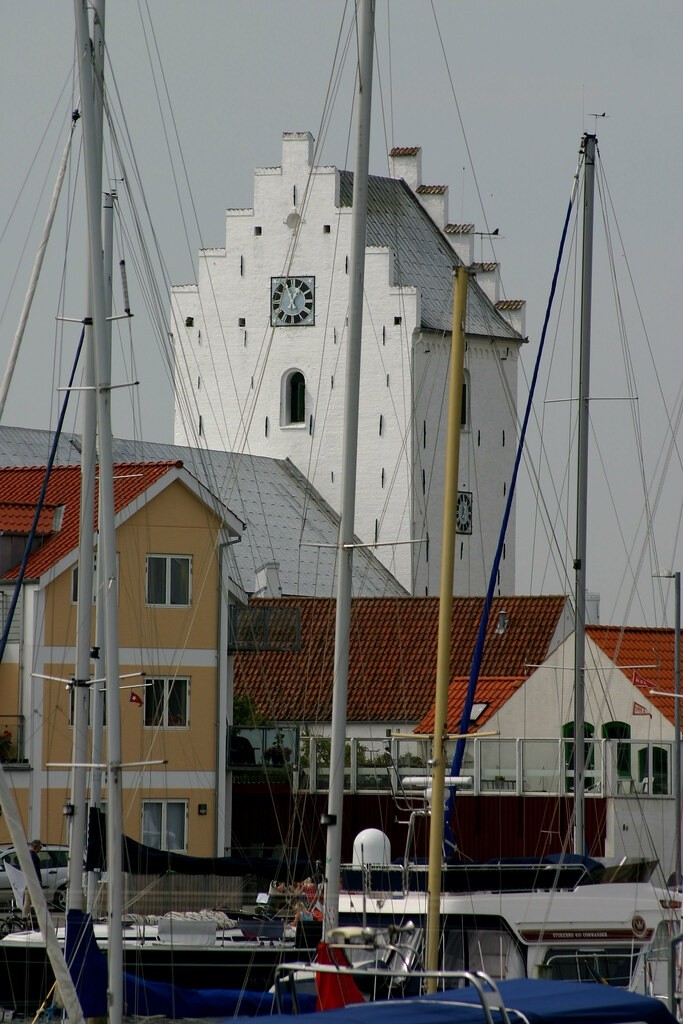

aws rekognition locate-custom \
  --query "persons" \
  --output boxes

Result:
[273,876,316,895]
[288,901,314,930]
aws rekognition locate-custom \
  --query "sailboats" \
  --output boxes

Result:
[0,0,683,1024]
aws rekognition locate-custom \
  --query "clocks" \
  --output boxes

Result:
[271,274,316,329]
[455,489,472,535]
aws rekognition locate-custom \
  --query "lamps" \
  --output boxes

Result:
[198,802,208,815]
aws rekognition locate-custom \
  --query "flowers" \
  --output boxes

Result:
[0,729,12,763]
